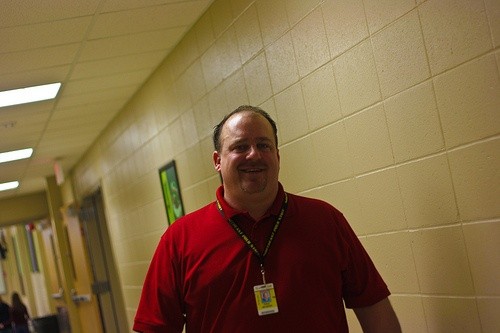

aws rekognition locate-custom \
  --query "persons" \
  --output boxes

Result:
[261,292,271,303]
[132,104,401,333]
[10,292,33,333]
[0,295,13,333]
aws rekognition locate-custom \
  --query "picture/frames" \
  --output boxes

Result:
[158,160,185,227]
